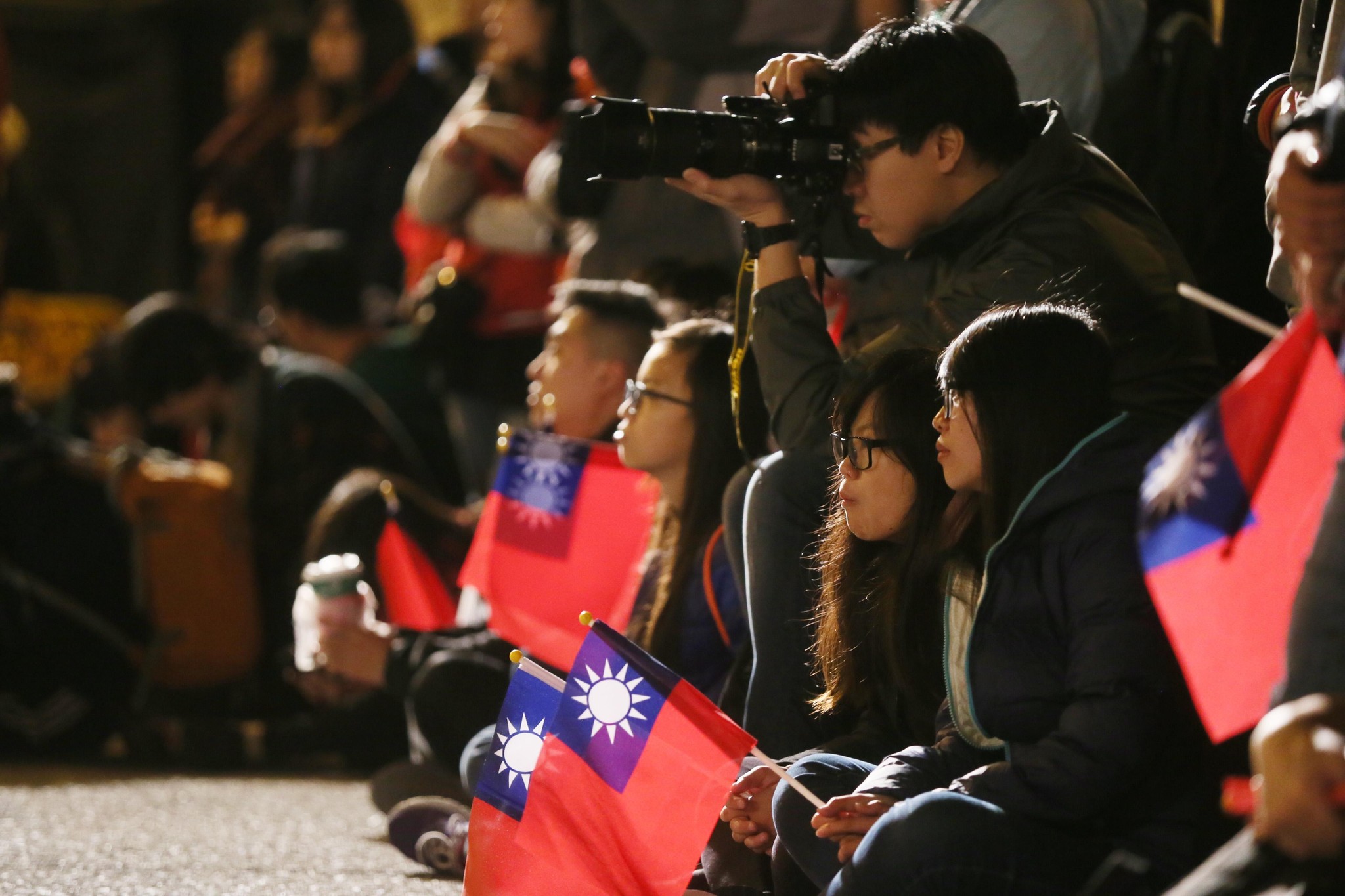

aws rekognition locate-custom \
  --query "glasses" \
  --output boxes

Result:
[851,136,904,179]
[627,380,694,411]
[945,380,976,418]
[832,430,898,470]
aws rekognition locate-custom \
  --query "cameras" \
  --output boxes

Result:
[580,78,846,196]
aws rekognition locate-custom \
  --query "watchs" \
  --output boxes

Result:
[741,220,801,260]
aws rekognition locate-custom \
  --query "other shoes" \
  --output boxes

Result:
[371,761,473,812]
[388,795,470,878]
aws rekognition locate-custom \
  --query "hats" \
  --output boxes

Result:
[120,290,249,408]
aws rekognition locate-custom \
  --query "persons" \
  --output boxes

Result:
[0,0,1345,896]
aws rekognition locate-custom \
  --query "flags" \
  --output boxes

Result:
[1135,306,1345,743]
[460,656,566,896]
[376,523,458,631]
[458,428,659,672]
[514,621,757,896]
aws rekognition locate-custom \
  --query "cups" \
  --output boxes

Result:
[304,553,362,602]
